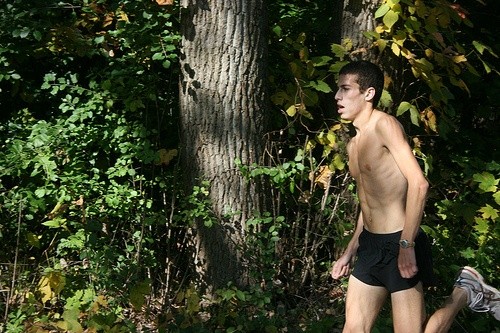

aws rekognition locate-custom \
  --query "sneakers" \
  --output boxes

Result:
[453,265,500,320]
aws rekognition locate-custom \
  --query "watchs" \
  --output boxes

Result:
[399,238,416,250]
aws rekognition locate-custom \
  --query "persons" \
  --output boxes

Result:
[330,59,500,333]
